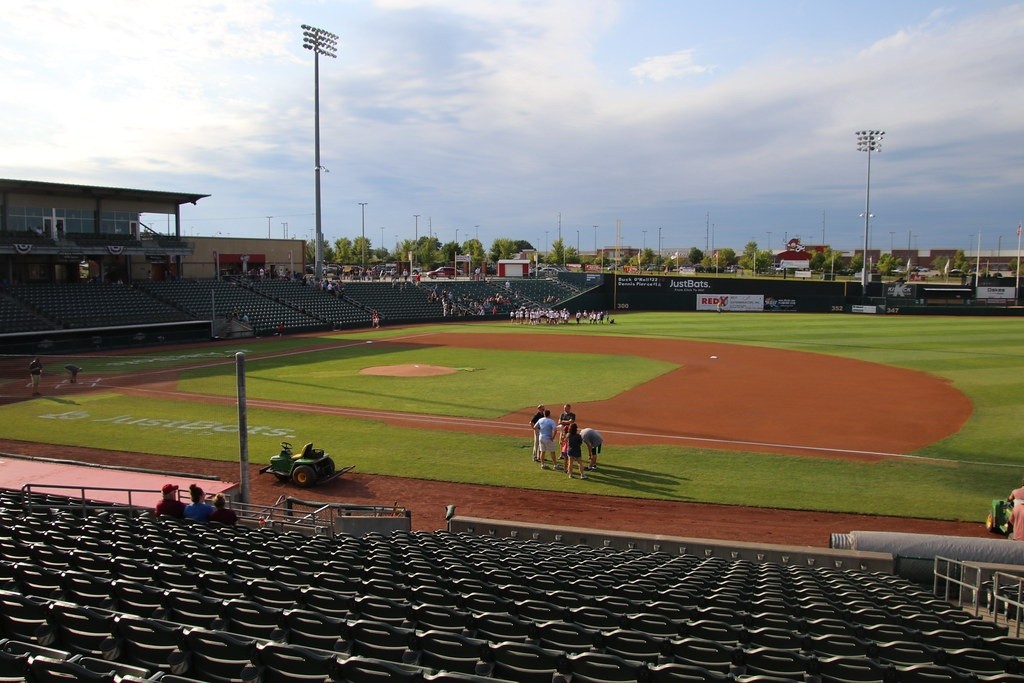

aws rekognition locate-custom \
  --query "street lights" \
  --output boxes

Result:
[381,215,480,249]
[301,25,339,283]
[267,216,287,239]
[359,202,368,265]
[856,130,885,292]
[537,210,1004,276]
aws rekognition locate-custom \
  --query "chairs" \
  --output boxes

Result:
[291,442,313,460]
[0,278,598,339]
[0,489,1024,683]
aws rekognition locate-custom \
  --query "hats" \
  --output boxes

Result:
[162,484,178,495]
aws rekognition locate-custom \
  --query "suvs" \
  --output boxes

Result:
[427,266,460,279]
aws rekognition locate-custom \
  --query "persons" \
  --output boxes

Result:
[529,267,534,279]
[505,279,510,294]
[232,309,250,324]
[576,428,603,470]
[29,357,45,398]
[1008,479,1024,510]
[475,267,481,275]
[245,265,421,297]
[183,484,214,523]
[63,364,83,383]
[372,311,381,328]
[208,493,239,524]
[157,484,186,518]
[278,321,284,337]
[576,310,609,324]
[529,403,576,473]
[565,423,588,479]
[1007,504,1024,542]
[427,287,570,325]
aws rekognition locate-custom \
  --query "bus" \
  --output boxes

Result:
[970,263,1014,278]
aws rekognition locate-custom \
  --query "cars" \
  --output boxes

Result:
[326,263,396,274]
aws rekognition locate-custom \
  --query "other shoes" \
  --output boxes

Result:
[563,470,568,473]
[541,465,550,469]
[581,475,588,479]
[592,465,597,469]
[569,476,574,478]
[32,392,40,396]
[553,465,564,470]
[583,467,592,472]
[534,460,540,463]
